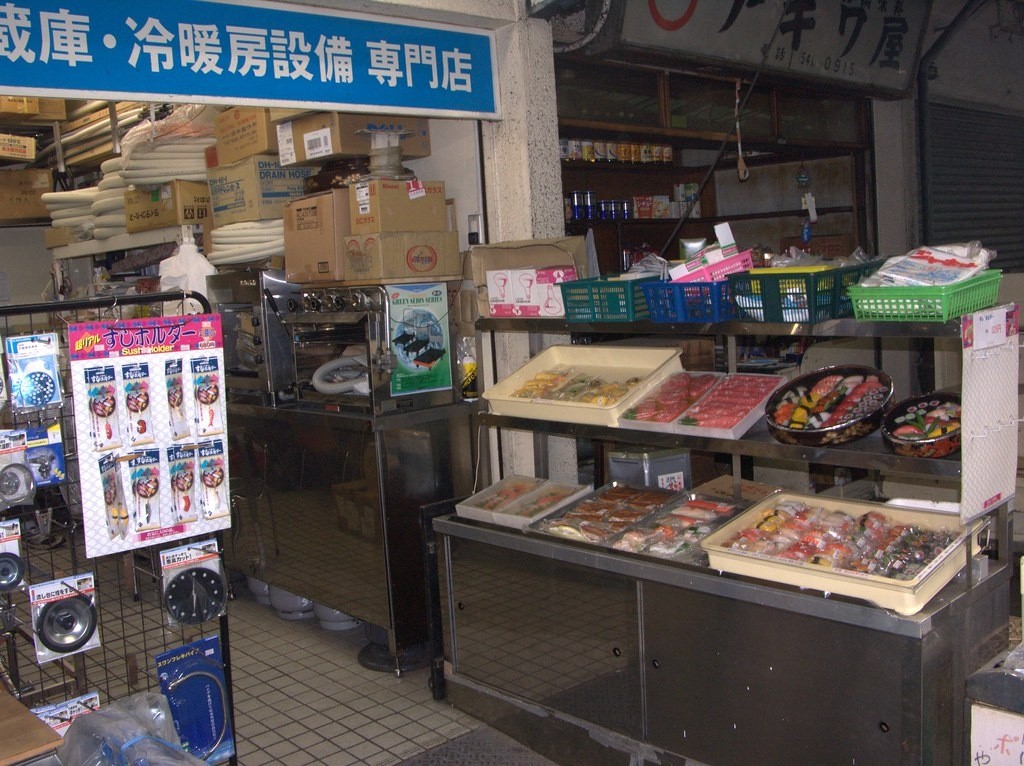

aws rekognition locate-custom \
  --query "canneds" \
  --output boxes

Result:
[558,137,673,165]
[564,190,630,222]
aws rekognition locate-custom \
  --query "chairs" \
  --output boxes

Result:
[231,439,280,562]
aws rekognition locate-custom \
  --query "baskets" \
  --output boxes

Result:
[553,274,670,323]
[724,259,886,324]
[639,278,746,323]
[846,269,1003,324]
[670,249,754,281]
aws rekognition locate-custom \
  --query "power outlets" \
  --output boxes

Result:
[467,215,481,245]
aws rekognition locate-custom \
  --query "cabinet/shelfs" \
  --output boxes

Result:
[434,318,1012,766]
[562,158,864,272]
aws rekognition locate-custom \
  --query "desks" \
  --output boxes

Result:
[221,392,474,677]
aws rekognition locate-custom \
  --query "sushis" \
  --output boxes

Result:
[720,501,958,580]
[888,399,960,441]
[773,373,889,430]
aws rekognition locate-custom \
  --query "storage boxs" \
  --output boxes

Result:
[455,447,992,616]
[0,95,68,121]
[0,169,53,219]
[469,233,1002,323]
[481,345,786,440]
[126,107,462,278]
[45,228,76,249]
[0,134,36,160]
[331,481,382,541]
